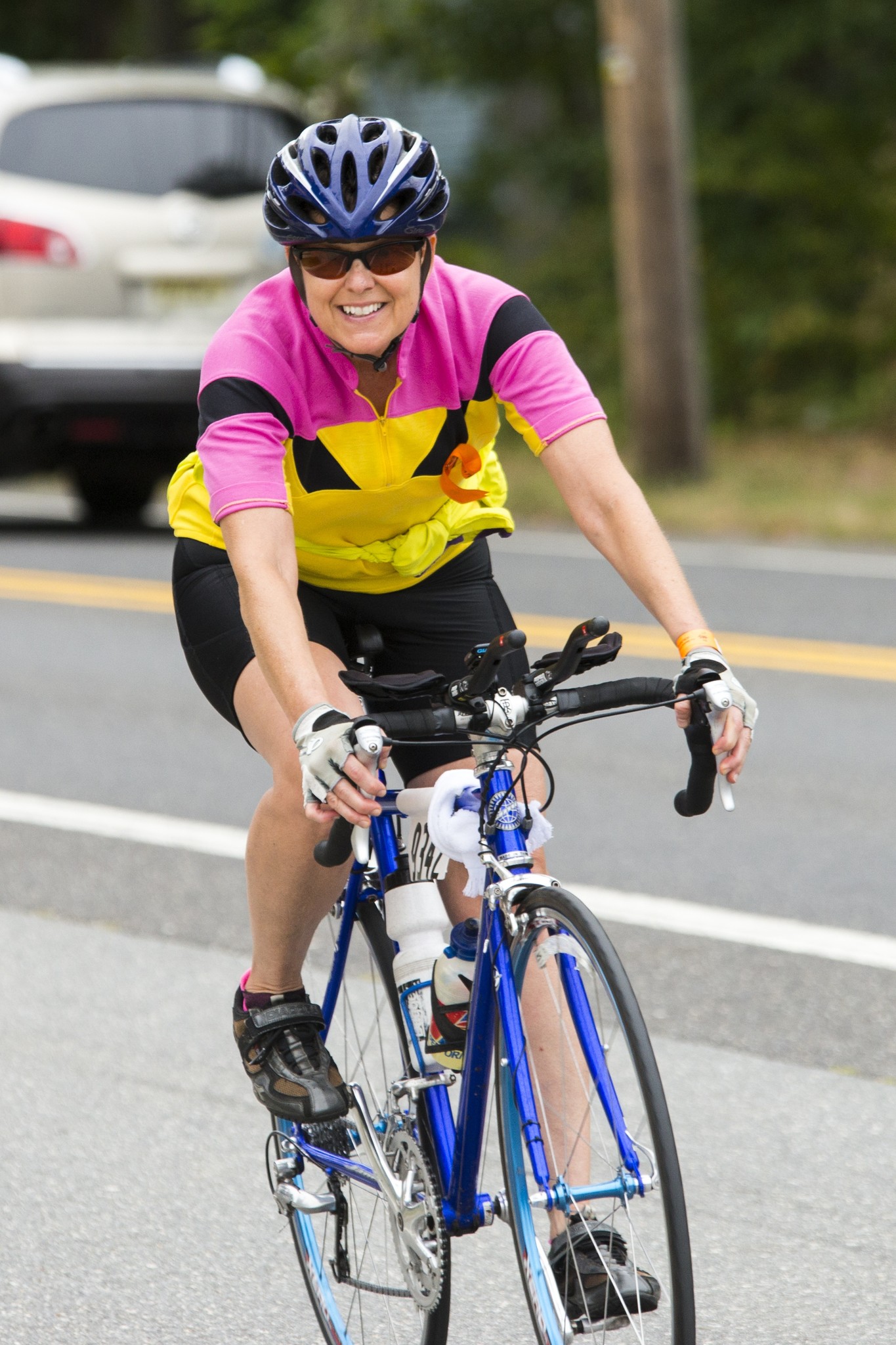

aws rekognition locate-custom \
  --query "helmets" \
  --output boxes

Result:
[263,111,452,249]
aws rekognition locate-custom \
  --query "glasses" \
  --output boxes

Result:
[294,231,435,280]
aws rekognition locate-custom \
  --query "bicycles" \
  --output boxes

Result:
[262,615,741,1344]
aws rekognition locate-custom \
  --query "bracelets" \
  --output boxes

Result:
[676,627,722,660]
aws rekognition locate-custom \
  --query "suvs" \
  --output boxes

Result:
[0,54,334,536]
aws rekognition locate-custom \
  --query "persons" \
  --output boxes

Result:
[166,113,756,1320]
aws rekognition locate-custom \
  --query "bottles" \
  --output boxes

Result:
[384,853,455,1074]
[425,917,482,1070]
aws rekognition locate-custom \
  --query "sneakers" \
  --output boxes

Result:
[226,971,357,1127]
[547,1210,655,1327]
